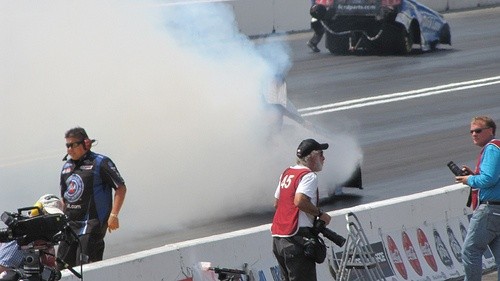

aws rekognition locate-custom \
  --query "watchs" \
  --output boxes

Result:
[317,209,324,217]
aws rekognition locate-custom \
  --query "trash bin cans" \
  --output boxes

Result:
[336,212,386,281]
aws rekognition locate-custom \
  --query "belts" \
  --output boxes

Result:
[480,201,500,205]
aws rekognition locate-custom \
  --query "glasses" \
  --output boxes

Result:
[66,140,82,148]
[470,127,490,133]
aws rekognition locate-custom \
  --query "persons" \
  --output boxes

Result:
[0,195,67,281]
[307,17,325,52]
[455,115,500,281]
[56,127,127,270]
[270,137,331,281]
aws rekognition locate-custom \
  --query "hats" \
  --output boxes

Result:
[296,138,329,159]
[40,193,65,215]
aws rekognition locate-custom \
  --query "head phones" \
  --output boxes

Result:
[84,139,96,150]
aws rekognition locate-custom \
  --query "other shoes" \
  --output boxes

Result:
[306,41,320,53]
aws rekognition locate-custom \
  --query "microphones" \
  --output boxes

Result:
[62,154,68,161]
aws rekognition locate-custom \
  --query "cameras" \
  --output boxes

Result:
[314,220,346,247]
[447,161,469,176]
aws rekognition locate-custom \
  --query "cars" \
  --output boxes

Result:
[310,0,452,55]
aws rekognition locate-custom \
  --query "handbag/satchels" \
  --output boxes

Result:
[303,236,327,263]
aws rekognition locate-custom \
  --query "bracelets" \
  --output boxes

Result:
[110,214,117,217]
